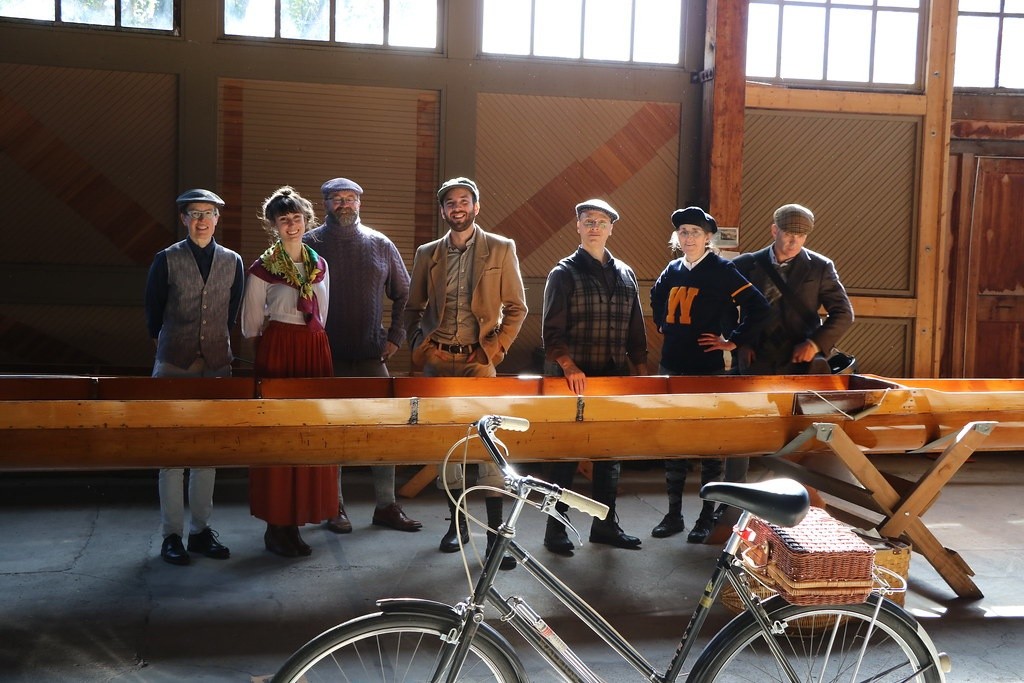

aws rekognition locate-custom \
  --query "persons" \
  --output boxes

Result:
[145,189,245,563]
[405,177,528,569]
[704,204,854,545]
[241,186,342,558]
[542,198,648,552]
[301,177,423,531]
[650,207,771,542]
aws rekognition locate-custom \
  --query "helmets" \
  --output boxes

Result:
[829,352,859,375]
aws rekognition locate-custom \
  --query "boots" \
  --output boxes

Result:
[440,489,469,550]
[544,501,575,554]
[586,501,641,548]
[485,495,518,566]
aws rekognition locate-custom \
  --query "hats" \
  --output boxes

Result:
[437,177,480,203]
[575,198,619,225]
[773,204,814,234]
[319,178,365,195]
[672,205,718,234]
[176,189,227,209]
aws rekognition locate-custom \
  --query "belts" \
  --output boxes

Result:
[430,339,480,356]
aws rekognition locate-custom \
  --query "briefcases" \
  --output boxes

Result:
[871,538,911,608]
[736,506,875,607]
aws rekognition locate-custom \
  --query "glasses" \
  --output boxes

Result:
[183,211,219,220]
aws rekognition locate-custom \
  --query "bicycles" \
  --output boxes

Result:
[270,415,951,683]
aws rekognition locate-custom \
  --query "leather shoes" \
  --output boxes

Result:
[653,514,686,537]
[263,522,313,558]
[688,514,719,542]
[162,533,191,566]
[373,504,421,528]
[189,527,232,558]
[327,503,352,532]
